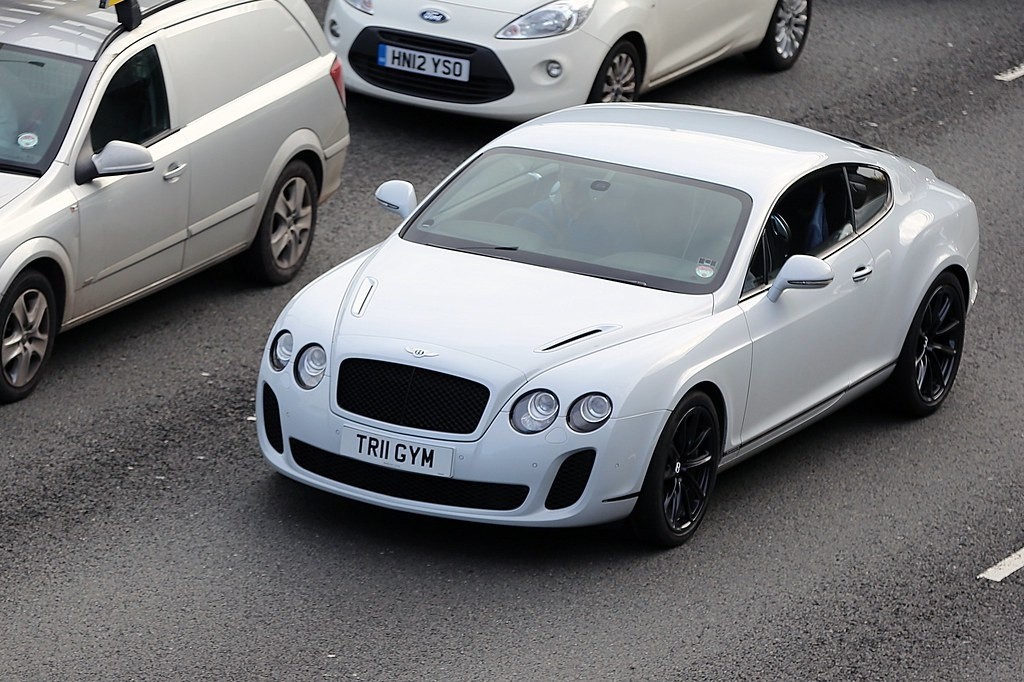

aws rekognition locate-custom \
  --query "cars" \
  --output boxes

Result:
[252,98,977,549]
[323,0,813,125]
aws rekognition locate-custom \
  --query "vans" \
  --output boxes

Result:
[0,0,354,403]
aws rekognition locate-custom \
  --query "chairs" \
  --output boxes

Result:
[92,65,153,148]
[767,185,829,264]
